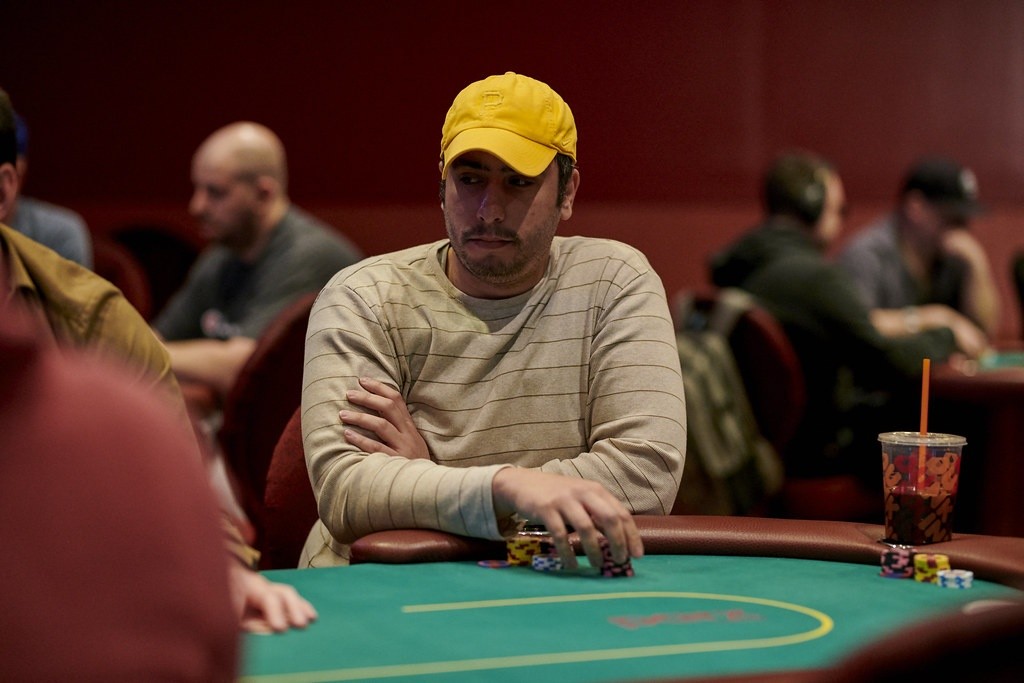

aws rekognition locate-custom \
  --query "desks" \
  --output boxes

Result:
[227,515,1024,683]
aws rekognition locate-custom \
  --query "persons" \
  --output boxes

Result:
[0,250,241,683]
[148,120,363,392]
[706,146,1023,526]
[300,69,689,568]
[0,58,318,633]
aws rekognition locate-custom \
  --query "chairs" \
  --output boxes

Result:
[921,335,1023,537]
[214,294,324,573]
[668,286,870,522]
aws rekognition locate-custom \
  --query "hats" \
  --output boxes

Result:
[439,70,579,177]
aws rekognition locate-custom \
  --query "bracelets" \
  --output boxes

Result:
[904,305,920,337]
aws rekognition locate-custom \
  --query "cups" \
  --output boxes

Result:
[878,431,967,546]
